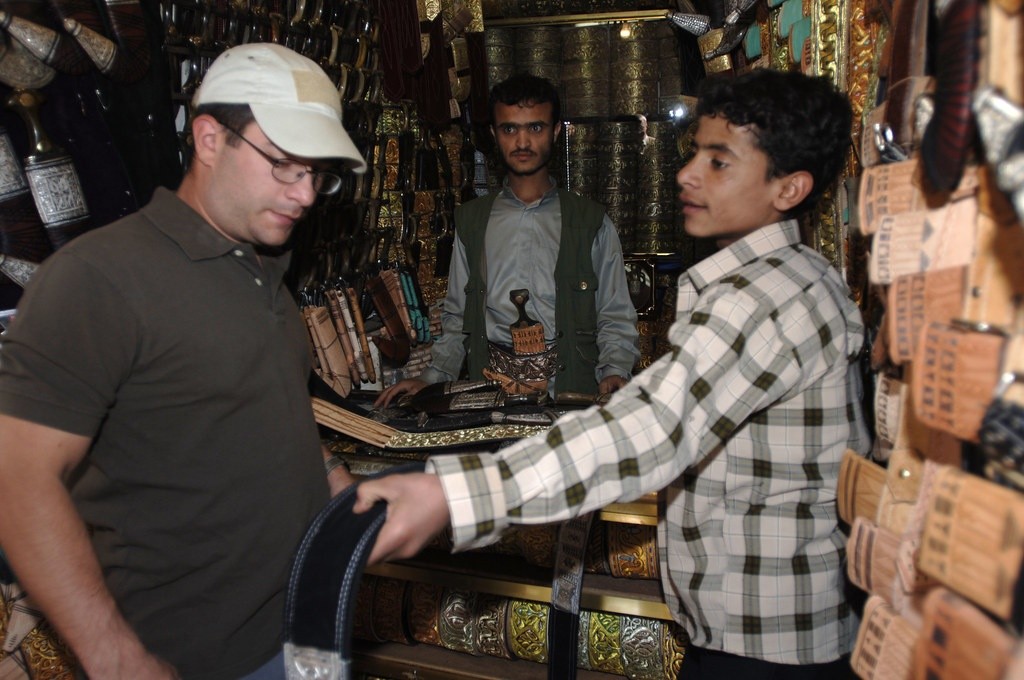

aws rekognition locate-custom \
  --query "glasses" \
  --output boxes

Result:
[218,121,343,195]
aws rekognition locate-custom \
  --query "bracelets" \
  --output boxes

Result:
[323,453,351,478]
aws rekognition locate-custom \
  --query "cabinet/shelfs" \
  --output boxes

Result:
[316,435,687,680]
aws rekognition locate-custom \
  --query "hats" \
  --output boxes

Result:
[191,43,366,174]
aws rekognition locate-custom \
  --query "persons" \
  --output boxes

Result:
[1,44,357,680]
[352,68,866,679]
[371,72,640,411]
